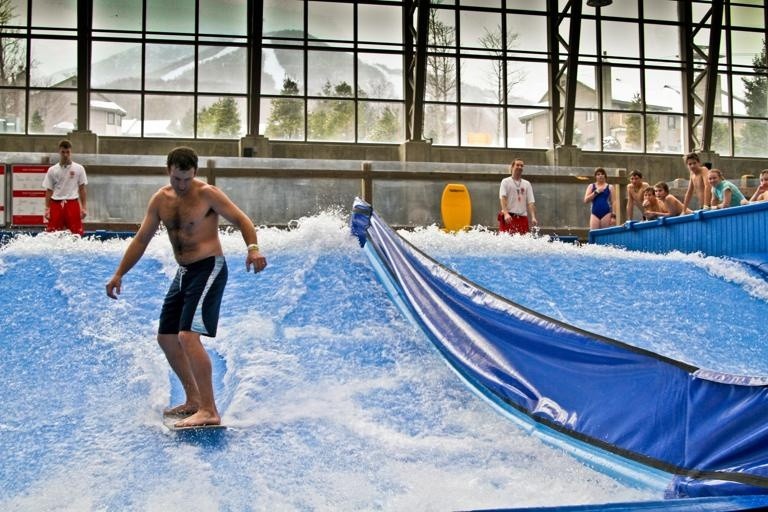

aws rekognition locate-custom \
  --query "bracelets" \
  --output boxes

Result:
[594,189,600,195]
[611,214,616,218]
[248,244,260,250]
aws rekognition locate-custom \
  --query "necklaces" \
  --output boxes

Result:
[513,178,521,187]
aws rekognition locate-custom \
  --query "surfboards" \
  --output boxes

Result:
[163,406,226,431]
[441,184,472,231]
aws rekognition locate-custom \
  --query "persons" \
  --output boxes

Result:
[42,140,88,236]
[584,167,616,230]
[498,158,538,236]
[106,146,267,427]
[626,153,768,222]
